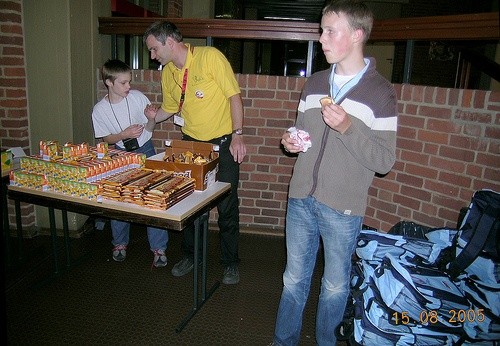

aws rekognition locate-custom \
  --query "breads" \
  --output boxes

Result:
[320,97,334,107]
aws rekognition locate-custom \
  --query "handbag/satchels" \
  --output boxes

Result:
[334,189,500,346]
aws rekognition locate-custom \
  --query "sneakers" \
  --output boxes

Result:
[171,257,201,276]
[153,249,167,267]
[222,265,239,284]
[112,245,126,261]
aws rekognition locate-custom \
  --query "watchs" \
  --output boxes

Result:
[232,129,242,135]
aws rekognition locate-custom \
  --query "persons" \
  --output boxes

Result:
[272,0,398,346]
[144,20,246,284]
[92,59,169,266]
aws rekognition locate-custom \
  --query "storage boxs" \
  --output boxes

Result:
[145,140,219,191]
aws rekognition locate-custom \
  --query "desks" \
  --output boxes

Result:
[7,182,232,332]
